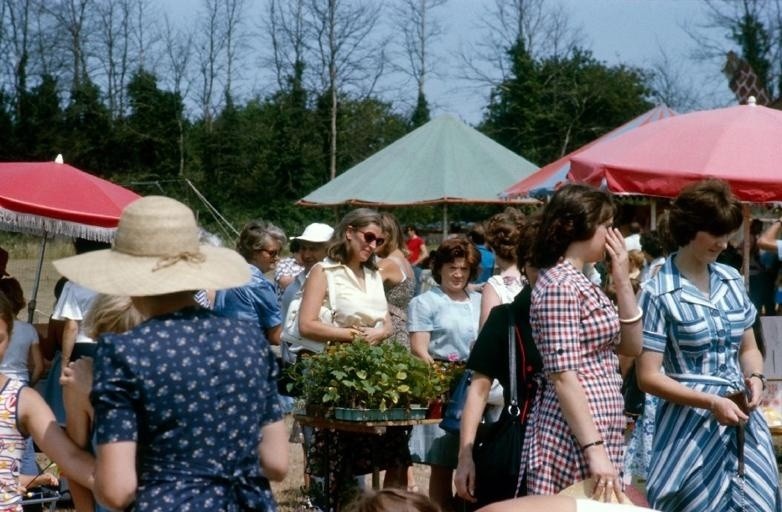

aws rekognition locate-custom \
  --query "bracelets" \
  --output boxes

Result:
[580,438,604,455]
[748,368,767,391]
[617,304,644,325]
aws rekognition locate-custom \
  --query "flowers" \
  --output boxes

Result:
[425,350,468,399]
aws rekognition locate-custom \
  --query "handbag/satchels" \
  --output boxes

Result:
[472,404,521,495]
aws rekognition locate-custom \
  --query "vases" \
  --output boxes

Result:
[425,396,443,419]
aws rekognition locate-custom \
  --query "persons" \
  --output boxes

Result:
[513,183,646,506]
[52,195,290,512]
[63,294,145,512]
[194,207,543,512]
[0,295,97,512]
[606,210,781,316]
[632,171,781,512]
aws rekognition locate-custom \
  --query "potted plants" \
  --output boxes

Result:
[283,329,434,423]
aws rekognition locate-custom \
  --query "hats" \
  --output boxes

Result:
[52,196,251,297]
[289,223,334,243]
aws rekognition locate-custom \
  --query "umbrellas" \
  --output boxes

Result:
[0,152,147,326]
[292,111,550,244]
[491,100,682,235]
[563,96,780,295]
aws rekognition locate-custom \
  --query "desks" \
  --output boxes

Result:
[291,415,444,512]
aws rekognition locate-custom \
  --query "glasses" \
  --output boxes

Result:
[359,230,384,245]
[263,249,276,256]
